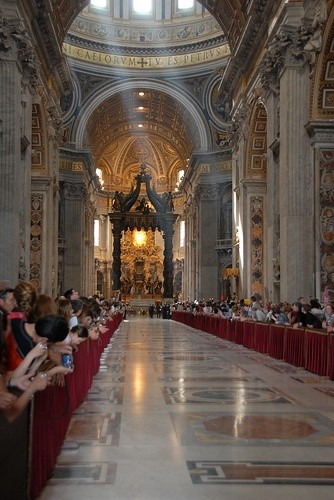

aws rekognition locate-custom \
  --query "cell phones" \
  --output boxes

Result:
[62,353,73,370]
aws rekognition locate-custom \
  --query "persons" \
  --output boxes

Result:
[0,274,133,425]
[147,287,334,337]
